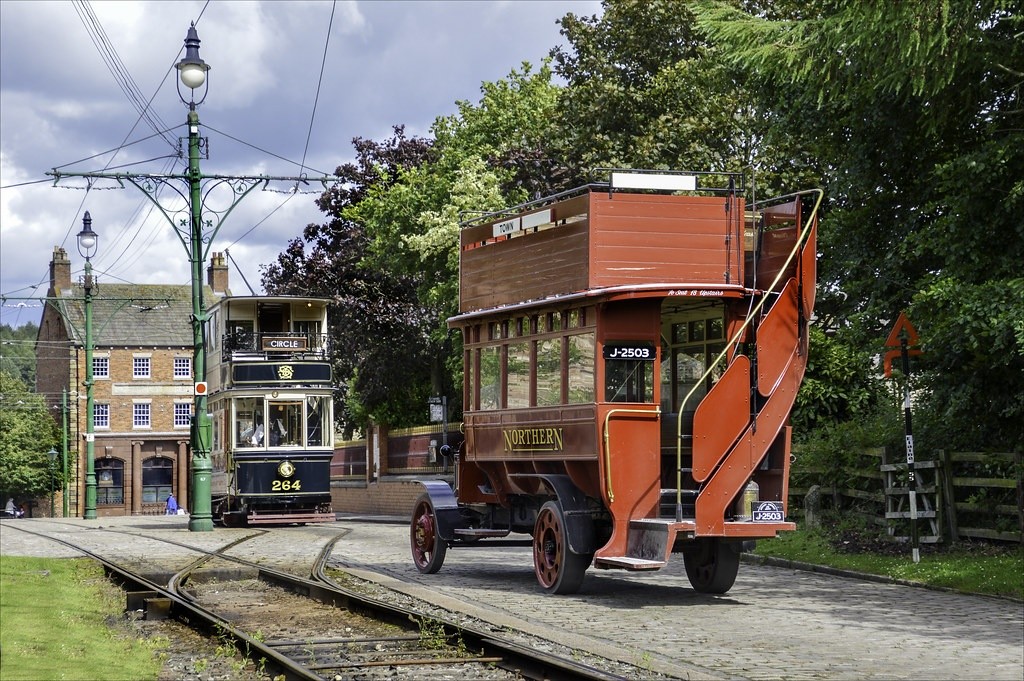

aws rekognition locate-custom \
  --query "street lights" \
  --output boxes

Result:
[75,210,101,520]
[174,16,215,531]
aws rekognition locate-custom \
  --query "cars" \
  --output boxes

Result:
[409,167,824,596]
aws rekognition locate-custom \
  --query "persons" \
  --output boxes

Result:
[5,498,24,518]
[269,419,277,446]
[165,493,175,515]
